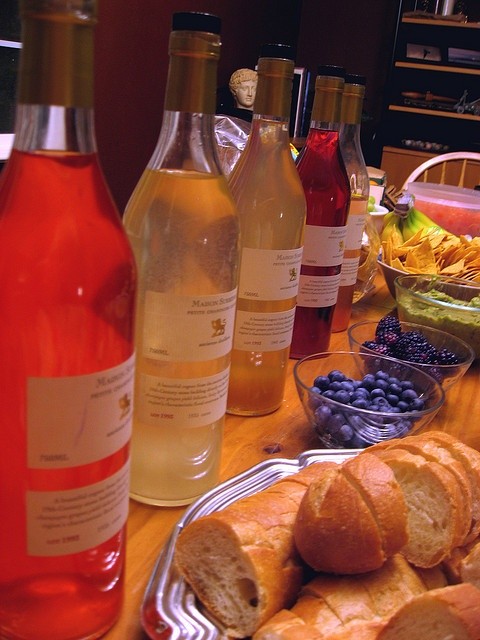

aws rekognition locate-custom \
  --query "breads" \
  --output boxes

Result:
[173,430,480,640]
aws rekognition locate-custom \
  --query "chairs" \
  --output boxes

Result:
[401,151,480,194]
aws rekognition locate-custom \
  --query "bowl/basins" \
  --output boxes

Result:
[392,272,479,365]
[294,350,446,451]
[347,318,477,398]
[377,260,479,302]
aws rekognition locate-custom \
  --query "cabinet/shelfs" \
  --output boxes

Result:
[380,0,480,197]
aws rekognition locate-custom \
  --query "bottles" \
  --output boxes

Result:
[121,8,243,509]
[227,41,309,417]
[329,72,382,336]
[1,2,139,637]
[288,60,335,363]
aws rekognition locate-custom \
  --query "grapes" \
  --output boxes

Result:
[367,195,376,213]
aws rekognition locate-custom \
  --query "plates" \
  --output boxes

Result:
[139,447,365,639]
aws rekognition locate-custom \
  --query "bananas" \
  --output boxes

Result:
[381,195,457,246]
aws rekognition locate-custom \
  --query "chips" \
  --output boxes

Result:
[381,224,480,286]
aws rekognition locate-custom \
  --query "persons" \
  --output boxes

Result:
[228,65,259,112]
